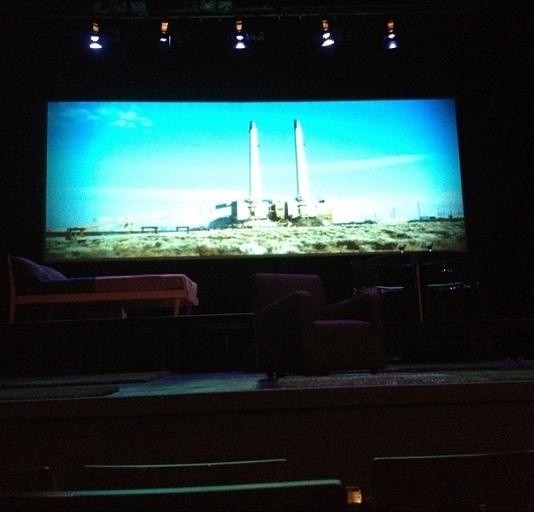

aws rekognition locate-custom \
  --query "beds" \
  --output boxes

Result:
[6,251,199,318]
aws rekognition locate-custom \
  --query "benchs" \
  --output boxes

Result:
[2,451,532,512]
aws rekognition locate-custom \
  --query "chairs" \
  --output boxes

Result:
[255,271,384,375]
